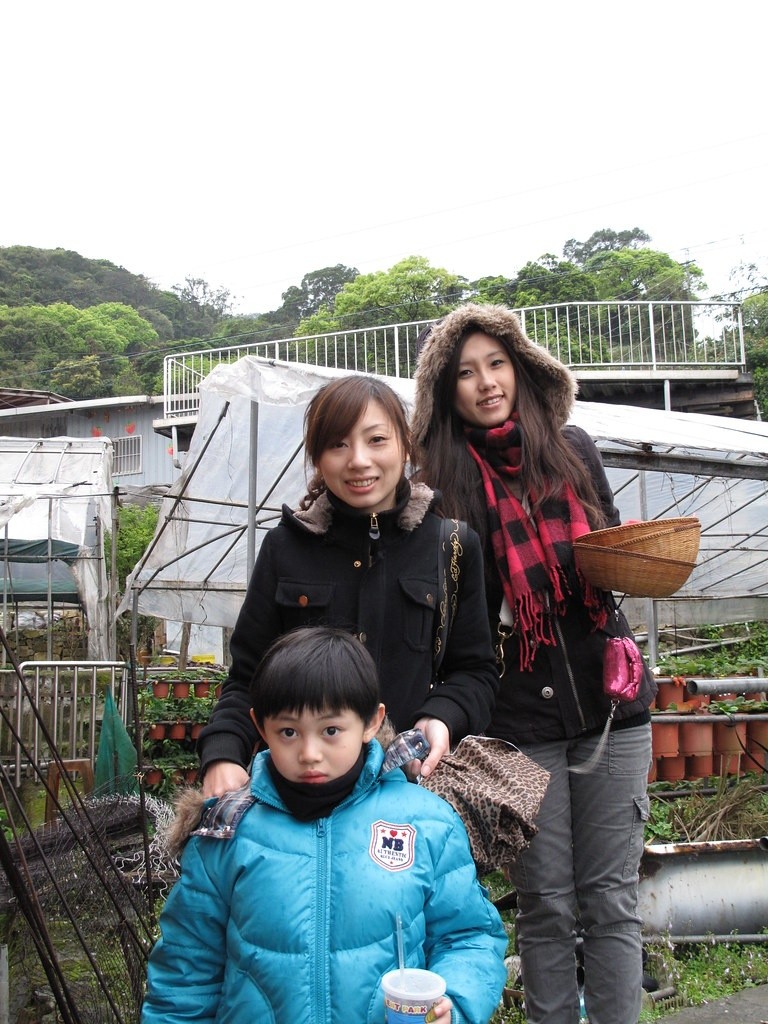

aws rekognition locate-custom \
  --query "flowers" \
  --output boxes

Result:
[655,654,686,687]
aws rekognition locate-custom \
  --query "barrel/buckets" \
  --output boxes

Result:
[160,656,178,665]
[192,654,215,664]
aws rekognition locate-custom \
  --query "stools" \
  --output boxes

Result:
[45,759,94,833]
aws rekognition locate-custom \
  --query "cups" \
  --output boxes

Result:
[382,968,447,1024]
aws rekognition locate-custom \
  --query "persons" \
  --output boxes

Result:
[410,301,660,1024]
[196,376,500,803]
[139,630,509,1024]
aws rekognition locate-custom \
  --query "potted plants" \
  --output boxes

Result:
[651,696,768,758]
[147,758,200,785]
[684,658,768,709]
[150,670,229,699]
[148,710,208,740]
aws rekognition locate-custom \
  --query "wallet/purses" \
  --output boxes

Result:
[604,635,647,706]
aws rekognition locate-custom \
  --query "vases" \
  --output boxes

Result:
[657,684,684,710]
[648,754,765,784]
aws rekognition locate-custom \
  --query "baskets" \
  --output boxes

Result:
[575,514,703,600]
